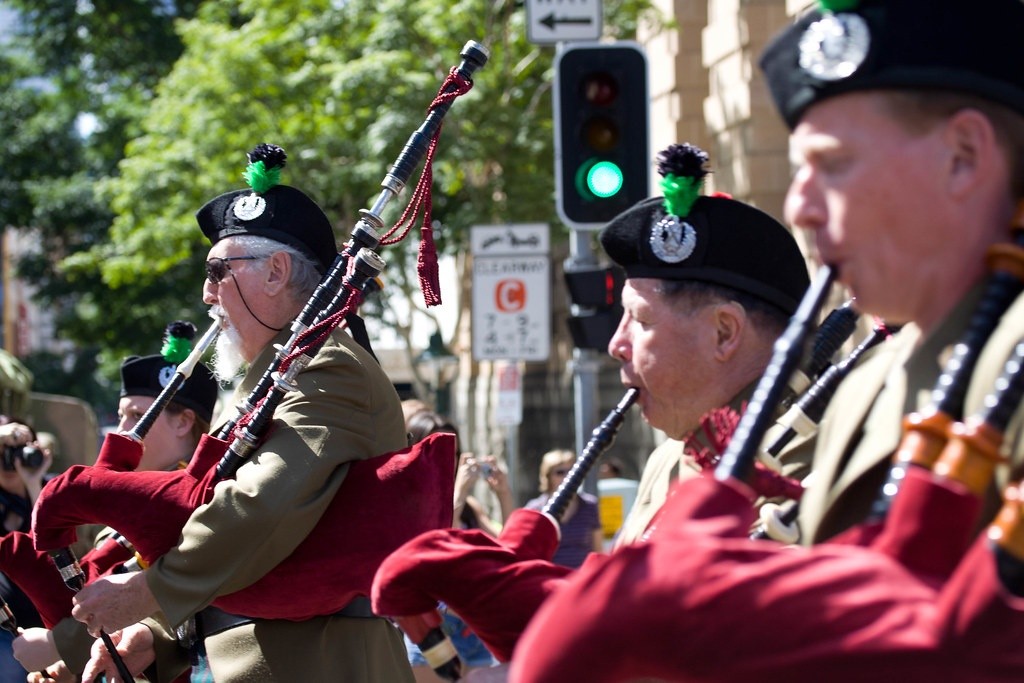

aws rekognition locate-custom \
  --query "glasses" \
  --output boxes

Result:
[205,254,270,283]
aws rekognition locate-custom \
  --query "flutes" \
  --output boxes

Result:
[513,245,1024,681]
[379,382,650,682]
[43,40,486,683]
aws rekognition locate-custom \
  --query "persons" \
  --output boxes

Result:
[2,1,1024,683]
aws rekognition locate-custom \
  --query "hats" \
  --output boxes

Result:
[757,1,1024,133]
[195,144,336,274]
[600,143,811,312]
[119,319,216,423]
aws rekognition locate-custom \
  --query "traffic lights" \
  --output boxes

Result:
[555,45,649,230]
